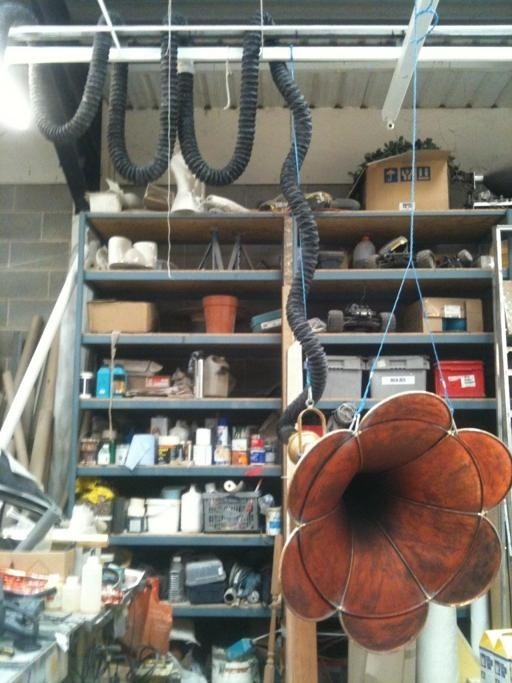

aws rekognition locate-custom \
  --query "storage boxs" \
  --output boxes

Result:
[88,299,155,333]
[304,356,367,398]
[403,297,484,333]
[1,550,75,585]
[346,148,450,211]
[184,554,227,603]
[432,361,486,399]
[367,355,431,399]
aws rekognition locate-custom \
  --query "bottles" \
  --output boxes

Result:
[351,234,375,269]
[111,360,127,397]
[43,574,61,609]
[265,507,281,537]
[61,574,79,613]
[80,556,103,615]
[168,555,185,603]
[97,428,116,463]
[95,359,110,399]
[217,417,229,449]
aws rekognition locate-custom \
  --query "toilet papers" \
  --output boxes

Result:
[224,480,245,494]
[416,602,457,683]
[196,428,211,446]
[193,445,212,467]
[470,594,488,654]
[133,242,158,267]
[109,237,131,262]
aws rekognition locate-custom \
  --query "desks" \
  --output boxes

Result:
[0,614,85,683]
[70,574,140,682]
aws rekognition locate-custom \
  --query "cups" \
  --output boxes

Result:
[108,235,159,267]
[249,436,266,465]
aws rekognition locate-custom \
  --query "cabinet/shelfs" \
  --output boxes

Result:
[66,210,512,682]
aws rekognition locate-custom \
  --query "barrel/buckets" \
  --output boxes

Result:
[180,484,203,535]
[145,498,181,534]
[205,354,229,398]
